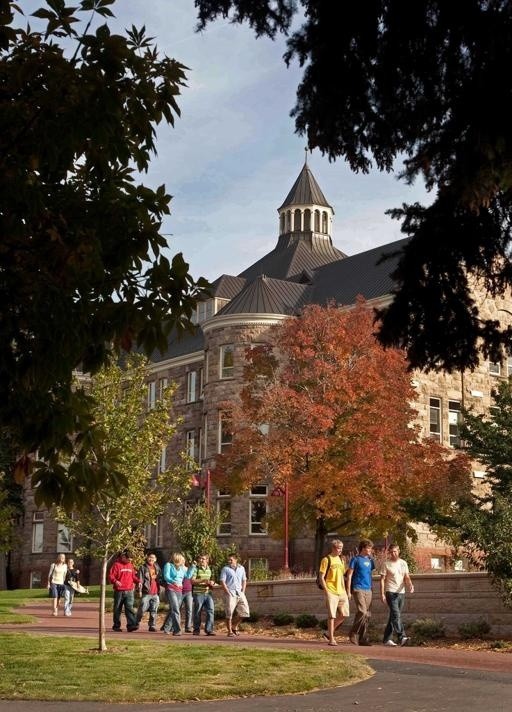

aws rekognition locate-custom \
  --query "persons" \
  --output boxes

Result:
[220,554,249,637]
[47,553,80,616]
[319,539,350,646]
[136,551,164,632]
[108,550,142,632]
[192,553,216,636]
[378,543,414,647]
[163,551,197,636]
[346,538,377,647]
[62,559,80,616]
[170,551,193,632]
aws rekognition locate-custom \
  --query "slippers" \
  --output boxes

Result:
[315,555,343,590]
[345,554,372,594]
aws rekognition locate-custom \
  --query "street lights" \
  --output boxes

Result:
[156,562,172,587]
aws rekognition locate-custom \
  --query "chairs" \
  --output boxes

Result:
[328,640,339,646]
[359,638,373,646]
[322,632,331,643]
[399,635,411,645]
[349,629,357,645]
[383,639,398,646]
[113,624,240,637]
